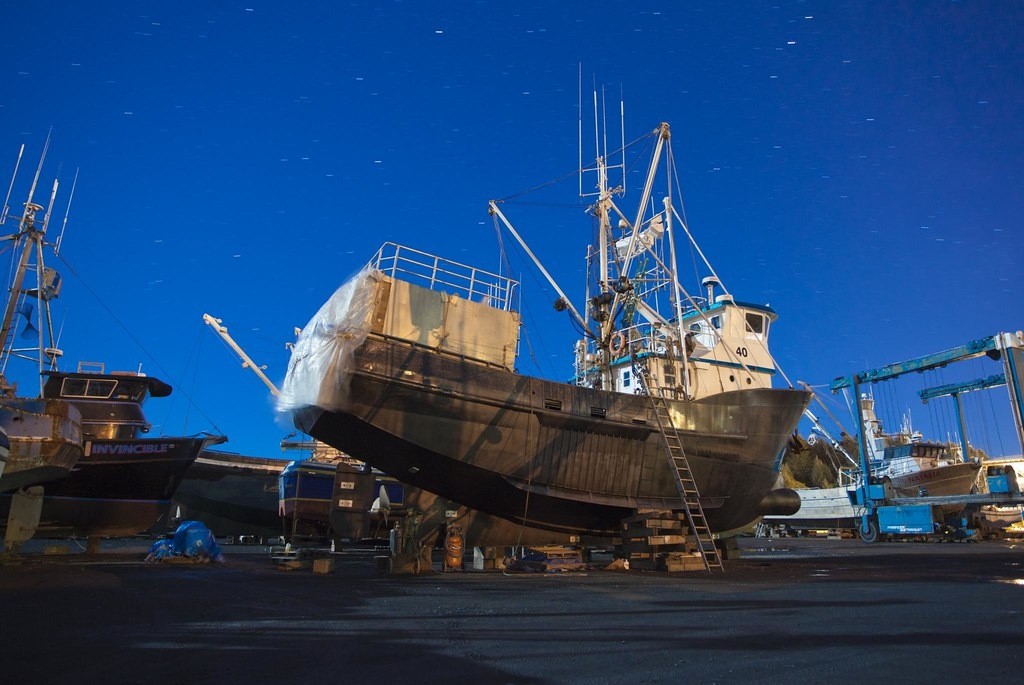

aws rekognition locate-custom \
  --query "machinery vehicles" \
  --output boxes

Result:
[830,330,1024,540]
[917,373,1007,514]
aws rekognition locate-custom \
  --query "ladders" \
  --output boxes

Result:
[634,372,726,575]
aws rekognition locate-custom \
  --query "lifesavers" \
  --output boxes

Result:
[609,331,625,355]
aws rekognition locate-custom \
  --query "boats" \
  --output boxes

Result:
[0,61,985,574]
